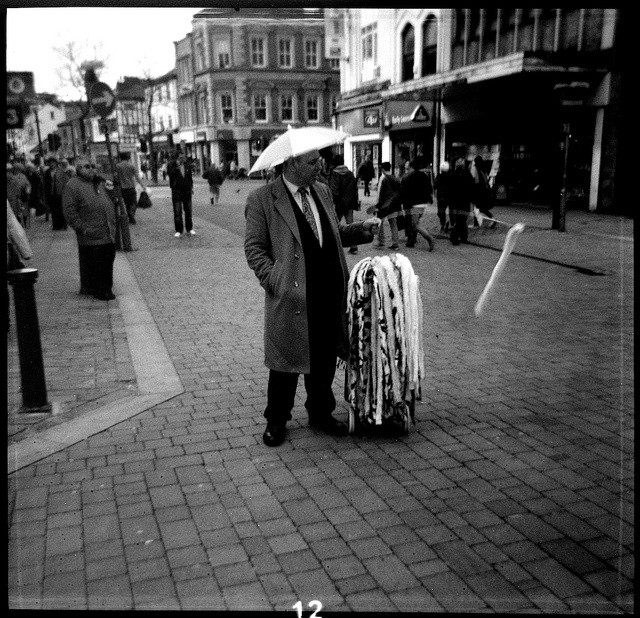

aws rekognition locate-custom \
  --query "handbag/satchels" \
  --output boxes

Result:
[396,211,407,230]
[137,191,151,208]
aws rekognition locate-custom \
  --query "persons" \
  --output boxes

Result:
[372,161,401,250]
[168,147,197,237]
[63,158,117,301]
[433,145,498,248]
[400,160,436,253]
[5,154,77,230]
[245,150,353,446]
[217,158,238,181]
[359,154,375,197]
[330,155,359,256]
[204,162,223,205]
[116,151,145,225]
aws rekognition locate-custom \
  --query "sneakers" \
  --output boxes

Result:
[80,287,90,294]
[488,218,495,228]
[190,230,195,235]
[89,290,116,300]
[406,241,414,248]
[388,246,399,250]
[468,225,478,229]
[349,248,358,254]
[429,238,436,251]
[210,197,214,204]
[373,242,385,249]
[175,232,181,238]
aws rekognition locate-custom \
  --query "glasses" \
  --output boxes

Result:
[85,164,95,168]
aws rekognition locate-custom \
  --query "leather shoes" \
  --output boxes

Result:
[310,416,348,438]
[263,417,286,446]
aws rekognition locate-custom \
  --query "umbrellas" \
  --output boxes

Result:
[247,125,352,177]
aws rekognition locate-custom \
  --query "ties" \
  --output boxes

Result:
[298,188,320,243]
